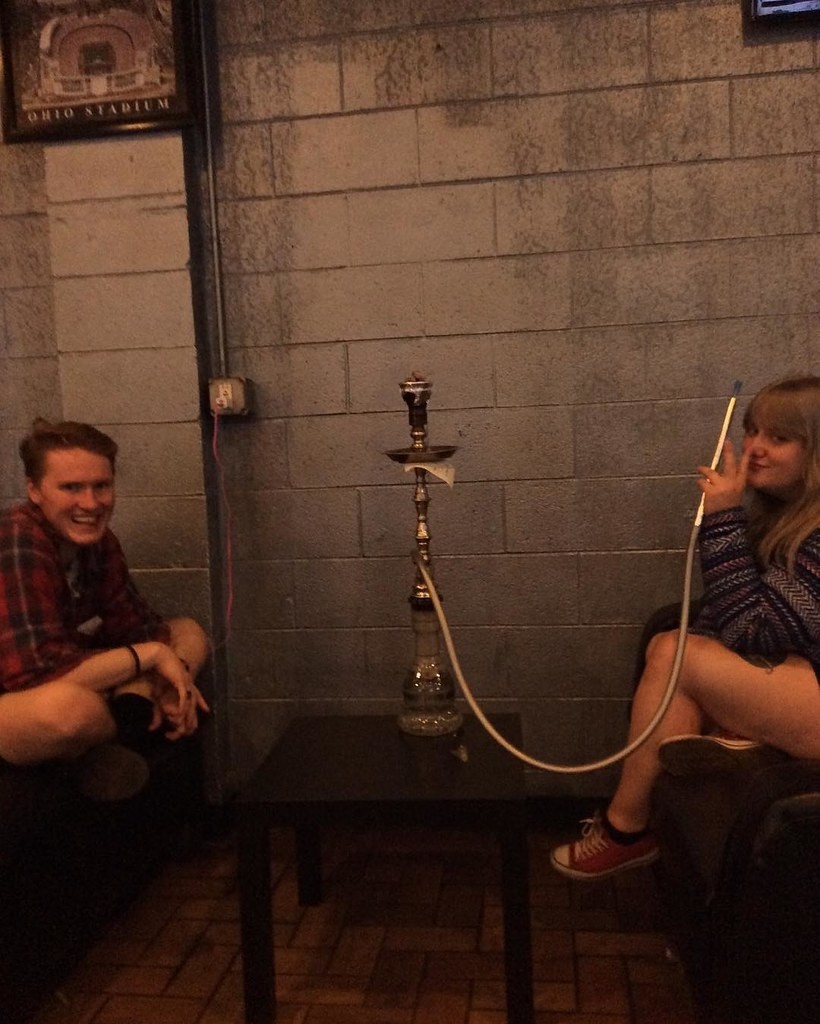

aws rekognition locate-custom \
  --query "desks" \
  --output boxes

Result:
[228,712,533,1024]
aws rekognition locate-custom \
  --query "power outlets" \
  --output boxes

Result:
[209,376,251,420]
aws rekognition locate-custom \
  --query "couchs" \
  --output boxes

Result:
[0,714,206,1024]
[626,601,820,1024]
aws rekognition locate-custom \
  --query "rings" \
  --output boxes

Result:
[186,690,192,697]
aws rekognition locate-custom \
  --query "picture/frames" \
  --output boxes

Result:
[741,0,820,32]
[0,0,181,145]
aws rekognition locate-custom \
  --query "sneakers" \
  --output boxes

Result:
[550,808,660,881]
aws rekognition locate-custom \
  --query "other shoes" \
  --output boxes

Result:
[64,741,150,804]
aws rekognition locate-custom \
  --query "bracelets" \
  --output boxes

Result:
[125,645,143,676]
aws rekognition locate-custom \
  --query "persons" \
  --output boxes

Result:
[0,413,211,801]
[549,374,820,878]
